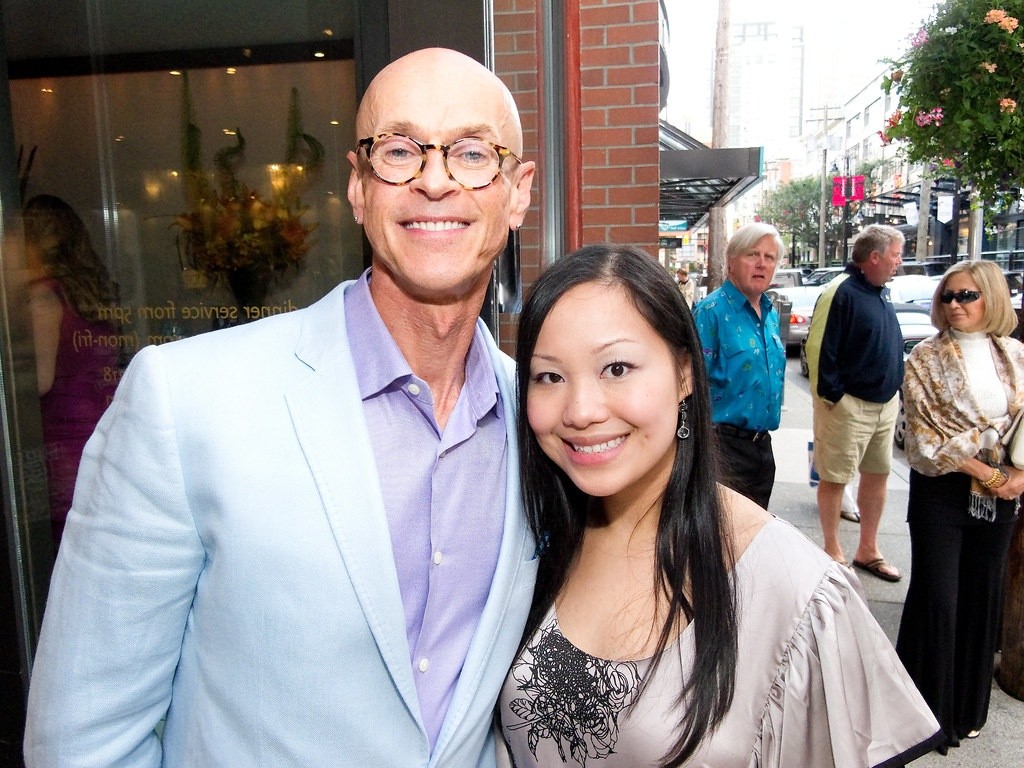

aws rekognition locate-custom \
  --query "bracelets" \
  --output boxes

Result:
[980,469,1001,488]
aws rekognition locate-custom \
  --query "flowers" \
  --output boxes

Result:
[132,82,326,326]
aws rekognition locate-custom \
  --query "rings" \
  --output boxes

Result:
[1008,496,1012,500]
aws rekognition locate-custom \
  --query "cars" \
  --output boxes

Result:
[693,261,1023,377]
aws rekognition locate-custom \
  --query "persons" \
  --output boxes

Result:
[22,45,570,768]
[0,194,128,548]
[495,245,942,768]
[670,223,1024,754]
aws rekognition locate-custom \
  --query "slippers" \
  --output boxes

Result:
[840,561,851,569]
[852,558,901,582]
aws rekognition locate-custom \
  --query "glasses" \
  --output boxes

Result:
[354,133,524,190]
[940,291,982,304]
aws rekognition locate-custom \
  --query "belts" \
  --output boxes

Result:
[716,424,768,442]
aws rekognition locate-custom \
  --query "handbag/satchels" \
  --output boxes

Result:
[808,442,820,487]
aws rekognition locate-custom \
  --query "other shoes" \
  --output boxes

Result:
[841,511,861,523]
